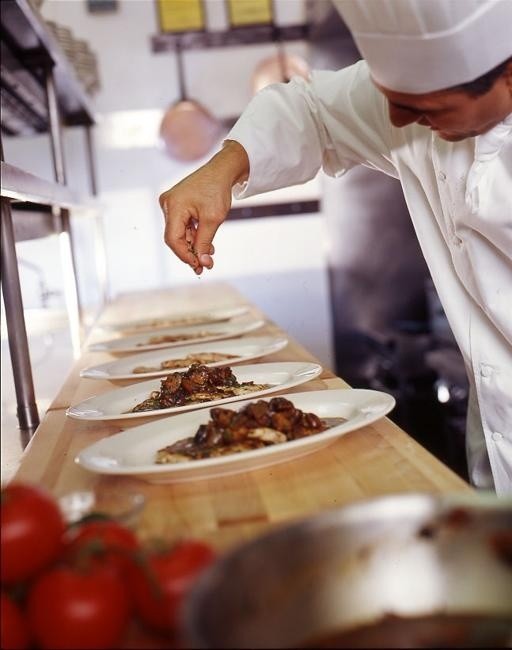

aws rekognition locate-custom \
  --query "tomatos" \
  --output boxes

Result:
[0,484,214,650]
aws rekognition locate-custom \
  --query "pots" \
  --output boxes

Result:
[161,55,316,167]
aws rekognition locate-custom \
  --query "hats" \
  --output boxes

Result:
[333,0,512,97]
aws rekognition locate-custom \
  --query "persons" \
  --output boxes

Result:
[157,1,512,496]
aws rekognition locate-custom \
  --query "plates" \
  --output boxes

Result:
[73,388,396,483]
[79,302,291,390]
[65,357,333,424]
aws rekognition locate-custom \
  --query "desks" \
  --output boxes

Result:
[6,274,484,561]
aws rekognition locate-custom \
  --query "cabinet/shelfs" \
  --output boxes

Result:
[150,21,325,221]
[0,0,111,476]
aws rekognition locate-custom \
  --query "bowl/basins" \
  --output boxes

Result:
[175,490,512,647]
